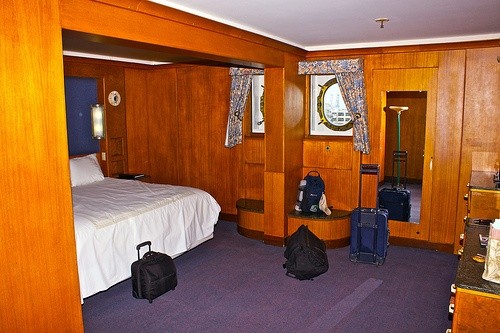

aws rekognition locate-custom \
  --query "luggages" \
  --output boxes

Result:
[378,148,411,221]
[348,162,390,265]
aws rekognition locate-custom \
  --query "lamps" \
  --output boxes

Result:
[90,104,107,140]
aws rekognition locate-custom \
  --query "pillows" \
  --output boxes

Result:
[69,153,104,187]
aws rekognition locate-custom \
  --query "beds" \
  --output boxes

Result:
[69,153,221,306]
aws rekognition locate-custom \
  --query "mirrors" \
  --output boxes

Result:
[376,89,429,224]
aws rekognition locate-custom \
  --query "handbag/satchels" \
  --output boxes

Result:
[294,170,332,216]
[129,240,179,303]
[282,224,329,281]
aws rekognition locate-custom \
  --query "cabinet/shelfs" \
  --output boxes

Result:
[446,170,500,333]
